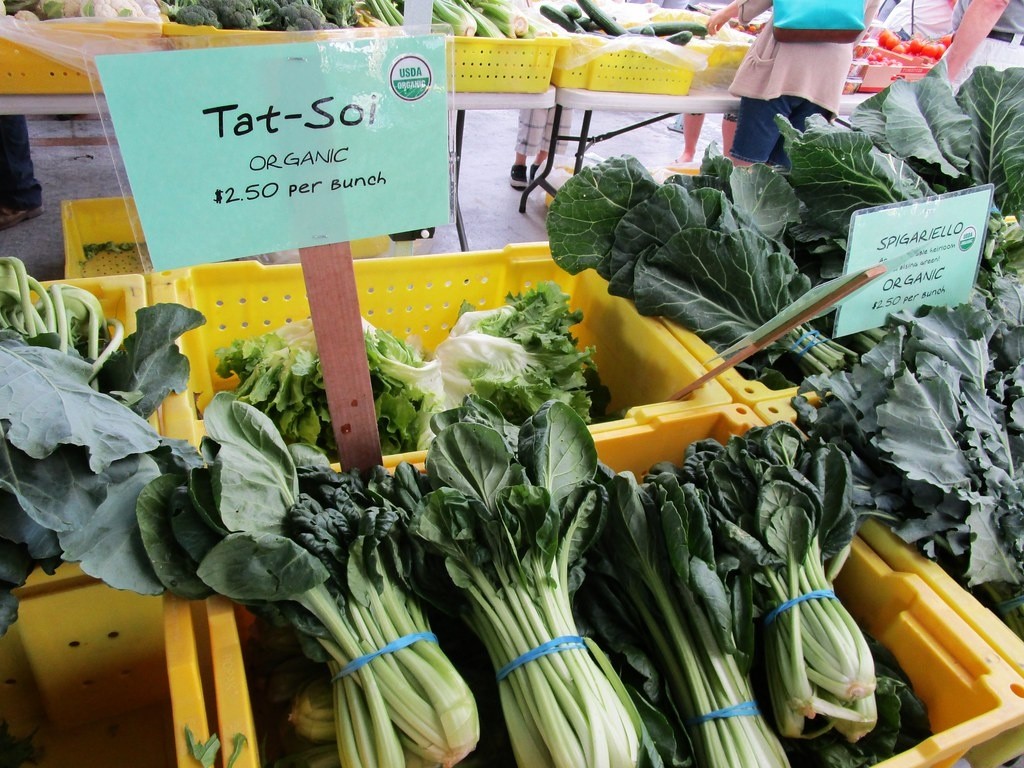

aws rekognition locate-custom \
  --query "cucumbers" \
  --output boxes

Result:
[540,0,708,46]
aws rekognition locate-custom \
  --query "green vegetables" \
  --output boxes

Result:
[544,57,1024,639]
[362,0,552,40]
[0,255,941,768]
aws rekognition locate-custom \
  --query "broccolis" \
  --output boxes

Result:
[0,0,358,31]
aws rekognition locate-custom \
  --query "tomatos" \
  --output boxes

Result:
[853,29,957,79]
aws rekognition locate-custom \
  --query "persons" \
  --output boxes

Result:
[666,0,1023,168]
[1,114,47,230]
[510,108,573,190]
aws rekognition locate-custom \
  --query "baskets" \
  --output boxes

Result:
[446,8,752,92]
[0,241,1024,768]
[61,195,389,279]
[0,5,404,94]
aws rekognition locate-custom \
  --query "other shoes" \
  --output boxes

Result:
[0,202,44,231]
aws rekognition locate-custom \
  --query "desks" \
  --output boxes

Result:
[0,94,108,115]
[519,88,878,213]
[448,84,557,252]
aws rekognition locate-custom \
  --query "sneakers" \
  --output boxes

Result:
[530,164,541,185]
[509,165,528,188]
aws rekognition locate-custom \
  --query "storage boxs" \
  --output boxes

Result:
[155,0,388,50]
[513,0,714,96]
[858,46,935,93]
[0,20,163,94]
[599,8,757,71]
[353,2,571,93]
[1,242,1024,767]
[61,195,153,279]
